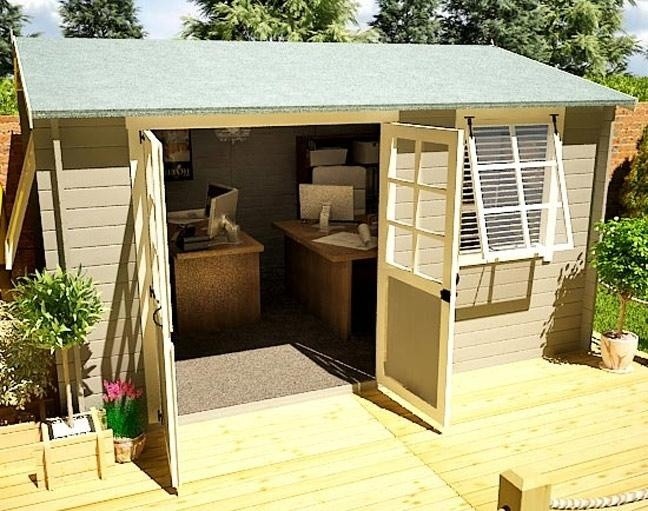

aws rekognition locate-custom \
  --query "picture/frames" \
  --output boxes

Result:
[152,130,194,181]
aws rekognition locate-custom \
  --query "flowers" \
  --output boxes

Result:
[101,379,148,438]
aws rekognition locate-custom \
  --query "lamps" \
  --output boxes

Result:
[214,129,251,147]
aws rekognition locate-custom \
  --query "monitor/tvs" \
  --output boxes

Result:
[299,184,355,232]
[204,183,238,220]
[207,190,242,246]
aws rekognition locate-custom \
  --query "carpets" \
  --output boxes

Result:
[175,343,376,413]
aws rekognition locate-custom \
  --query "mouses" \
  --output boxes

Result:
[200,226,207,230]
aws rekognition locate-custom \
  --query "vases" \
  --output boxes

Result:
[114,433,146,463]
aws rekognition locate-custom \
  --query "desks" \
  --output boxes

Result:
[168,225,264,337]
[273,212,378,351]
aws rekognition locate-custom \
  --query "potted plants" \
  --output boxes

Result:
[588,215,647,374]
[7,264,104,491]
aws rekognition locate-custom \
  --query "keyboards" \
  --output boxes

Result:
[175,226,196,250]
[310,222,358,230]
